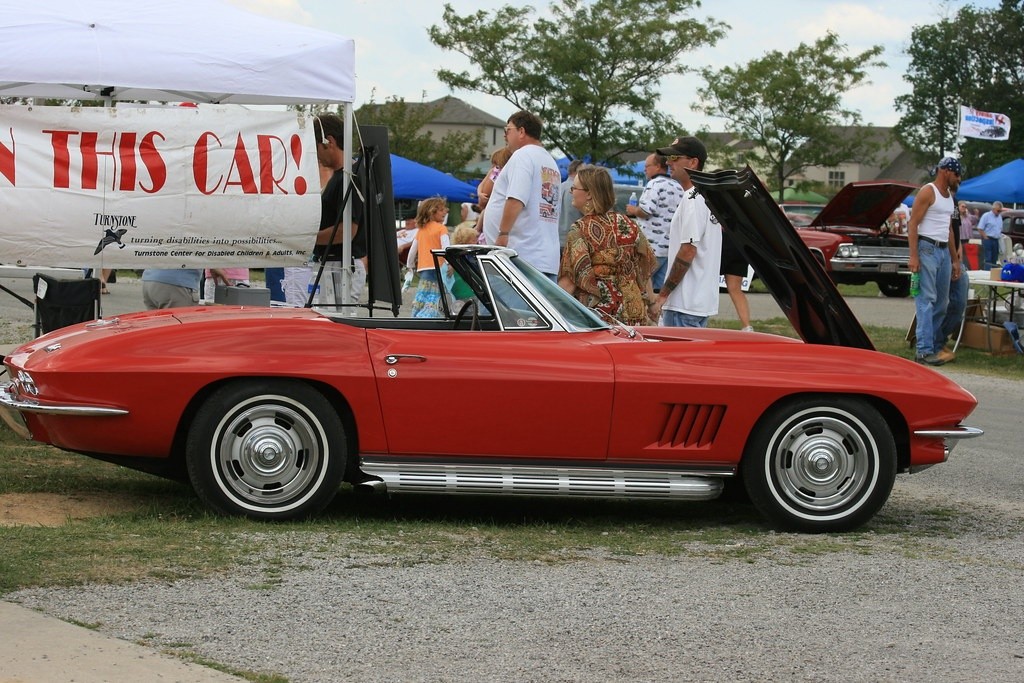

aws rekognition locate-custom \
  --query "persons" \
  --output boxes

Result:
[626,135,722,328]
[976,201,1003,270]
[720,233,754,332]
[99,268,111,295]
[478,109,560,311]
[940,183,969,354]
[1012,243,1024,258]
[907,156,962,365]
[956,202,972,271]
[558,159,659,326]
[888,203,910,234]
[205,269,250,288]
[1001,233,1013,263]
[284,111,365,315]
[143,269,202,310]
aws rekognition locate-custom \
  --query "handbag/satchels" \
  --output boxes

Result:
[401,267,414,295]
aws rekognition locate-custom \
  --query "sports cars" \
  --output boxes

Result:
[0,163,985,533]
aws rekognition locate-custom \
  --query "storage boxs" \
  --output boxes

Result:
[214,283,271,307]
[961,321,1013,350]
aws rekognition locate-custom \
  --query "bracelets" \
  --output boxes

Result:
[498,231,509,236]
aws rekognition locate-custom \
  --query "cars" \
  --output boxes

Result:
[776,180,923,297]
[952,201,1024,247]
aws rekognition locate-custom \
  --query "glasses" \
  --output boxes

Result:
[995,208,1002,213]
[569,184,588,193]
[663,155,693,161]
[948,169,960,178]
[504,126,522,135]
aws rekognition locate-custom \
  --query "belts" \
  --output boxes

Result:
[918,235,948,249]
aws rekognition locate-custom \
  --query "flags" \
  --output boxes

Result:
[959,105,1011,141]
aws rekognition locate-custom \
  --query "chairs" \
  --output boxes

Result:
[32,272,102,338]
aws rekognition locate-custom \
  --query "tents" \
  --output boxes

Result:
[954,159,1024,209]
[389,152,478,230]
[0,0,356,317]
[554,152,670,187]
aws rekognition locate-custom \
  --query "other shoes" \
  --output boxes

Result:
[471,204,481,213]
[915,345,956,365]
[741,325,754,333]
[102,282,110,294]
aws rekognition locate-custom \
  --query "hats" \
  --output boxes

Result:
[656,136,707,163]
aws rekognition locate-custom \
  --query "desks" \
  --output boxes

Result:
[953,279,1024,353]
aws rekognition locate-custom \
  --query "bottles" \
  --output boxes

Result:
[910,271,920,296]
[307,271,320,308]
